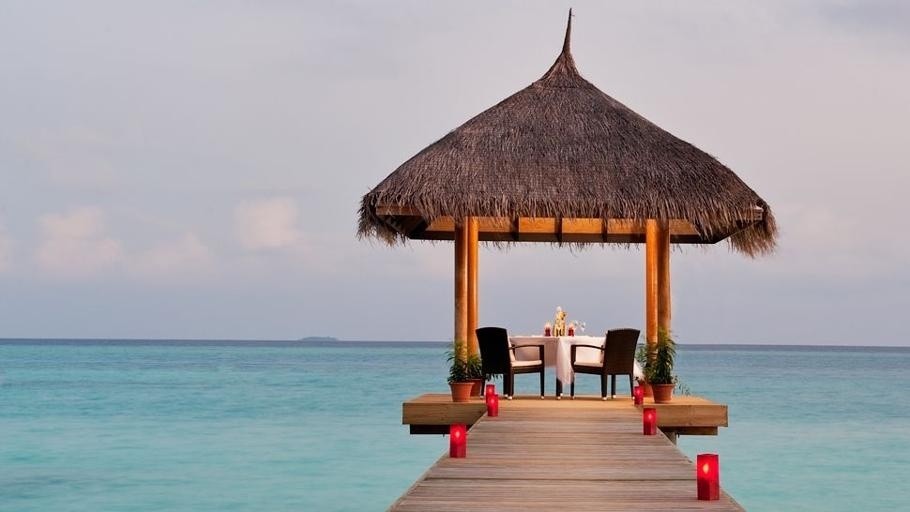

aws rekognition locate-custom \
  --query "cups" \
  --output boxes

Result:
[697,453,719,499]
[643,407,657,435]
[486,383,498,416]
[450,422,466,457]
[634,385,644,405]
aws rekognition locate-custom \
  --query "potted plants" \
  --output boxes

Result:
[632,339,657,397]
[443,343,477,403]
[649,327,679,403]
[467,349,501,397]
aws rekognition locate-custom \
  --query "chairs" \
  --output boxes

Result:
[569,326,642,401]
[474,326,547,401]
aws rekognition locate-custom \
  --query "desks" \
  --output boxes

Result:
[509,333,609,400]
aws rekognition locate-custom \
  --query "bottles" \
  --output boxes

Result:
[544,323,551,336]
[568,324,575,336]
